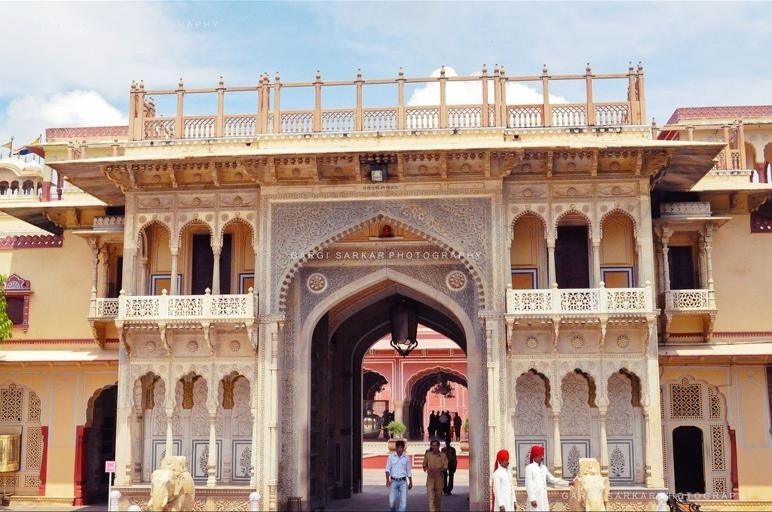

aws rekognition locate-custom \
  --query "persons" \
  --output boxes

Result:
[491,449,518,511]
[385,440,412,512]
[381,409,395,439]
[441,438,457,495]
[427,409,462,441]
[422,439,448,512]
[524,445,575,512]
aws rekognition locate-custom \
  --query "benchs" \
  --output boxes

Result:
[13,137,40,156]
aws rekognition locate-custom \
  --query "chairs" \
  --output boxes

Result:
[442,490,453,496]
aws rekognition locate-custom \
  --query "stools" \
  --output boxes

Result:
[390,476,407,481]
